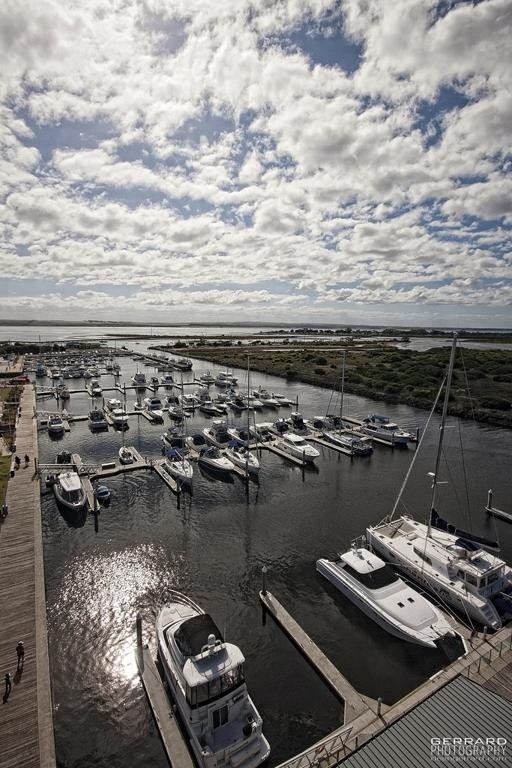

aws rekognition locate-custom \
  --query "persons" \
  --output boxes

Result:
[4,672,12,691]
[14,455,22,470]
[24,453,30,467]
[16,640,26,663]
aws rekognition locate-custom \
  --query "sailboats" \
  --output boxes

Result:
[225,353,260,474]
[321,350,373,458]
[164,380,195,486]
[363,332,512,633]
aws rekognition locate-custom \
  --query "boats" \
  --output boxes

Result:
[52,470,88,512]
[38,353,295,434]
[199,447,236,475]
[362,413,417,444]
[132,583,271,768]
[274,431,320,463]
[315,543,456,650]
